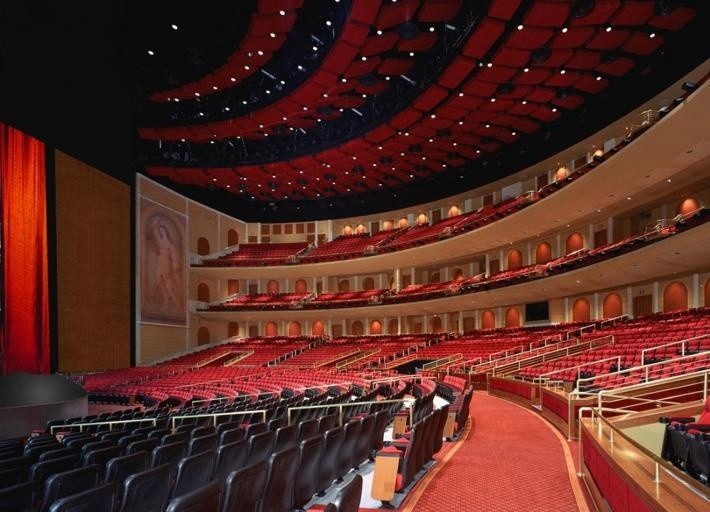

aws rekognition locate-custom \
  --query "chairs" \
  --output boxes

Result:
[0,330,472,511]
[375,305,710,421]
[198,190,534,266]
[217,226,652,316]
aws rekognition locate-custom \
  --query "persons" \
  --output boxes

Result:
[152,216,184,312]
